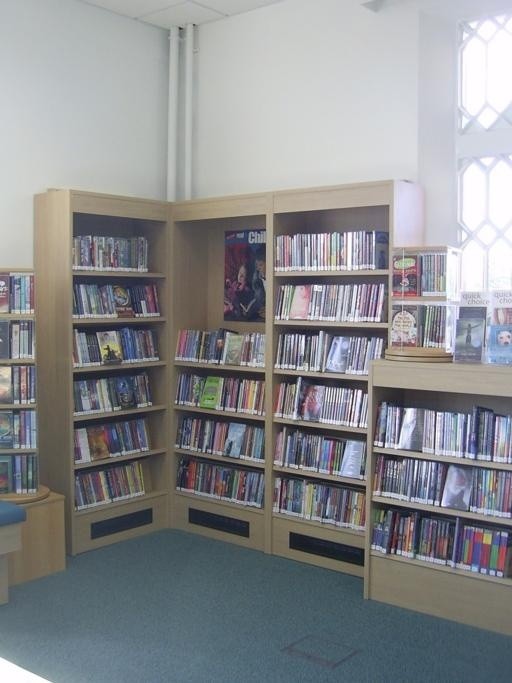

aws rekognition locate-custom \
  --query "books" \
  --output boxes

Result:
[175,328,266,508]
[73,236,162,508]
[390,253,512,364]
[274,230,388,533]
[0,273,38,493]
[372,401,512,577]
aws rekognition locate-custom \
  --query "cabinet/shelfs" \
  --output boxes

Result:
[364,358,512,637]
[173,179,428,578]
[16,491,65,586]
[1,268,50,505]
[34,190,174,556]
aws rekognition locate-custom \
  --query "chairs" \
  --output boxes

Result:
[1,501,26,603]
